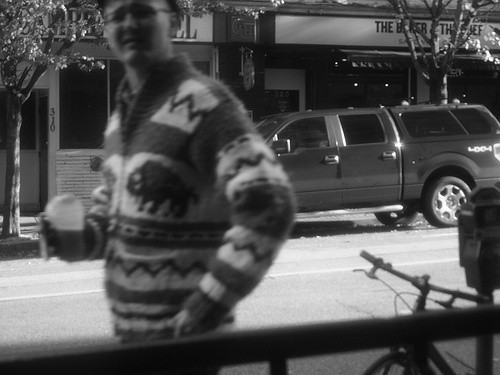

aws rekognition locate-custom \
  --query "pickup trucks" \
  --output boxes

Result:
[249,103,500,228]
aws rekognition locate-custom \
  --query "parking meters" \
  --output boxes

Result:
[457,182,500,375]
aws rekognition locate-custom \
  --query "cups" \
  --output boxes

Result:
[38,193,85,263]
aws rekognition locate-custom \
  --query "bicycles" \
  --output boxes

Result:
[353,249,500,375]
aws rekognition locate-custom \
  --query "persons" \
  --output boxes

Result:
[37,0,296,375]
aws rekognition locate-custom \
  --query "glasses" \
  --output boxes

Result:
[99,7,174,26]
